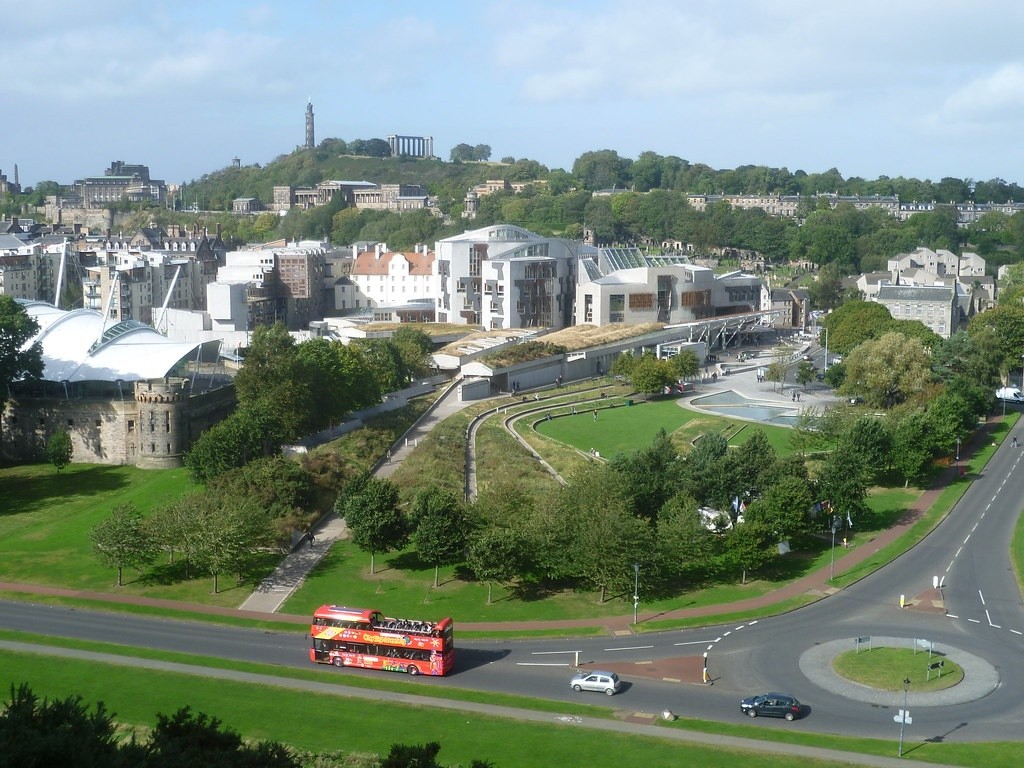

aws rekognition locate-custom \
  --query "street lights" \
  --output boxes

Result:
[899,676,911,757]
[831,525,836,581]
[634,562,639,624]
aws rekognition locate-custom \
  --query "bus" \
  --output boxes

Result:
[305,605,455,676]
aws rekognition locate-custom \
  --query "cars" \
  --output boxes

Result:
[570,671,621,696]
[803,355,813,360]
[740,692,801,721]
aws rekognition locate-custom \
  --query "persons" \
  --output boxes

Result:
[593,409,598,422]
[317,616,435,661]
[1010,436,1018,448]
[513,380,515,390]
[545,412,552,422]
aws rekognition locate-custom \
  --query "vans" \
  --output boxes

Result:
[996,387,1024,403]
